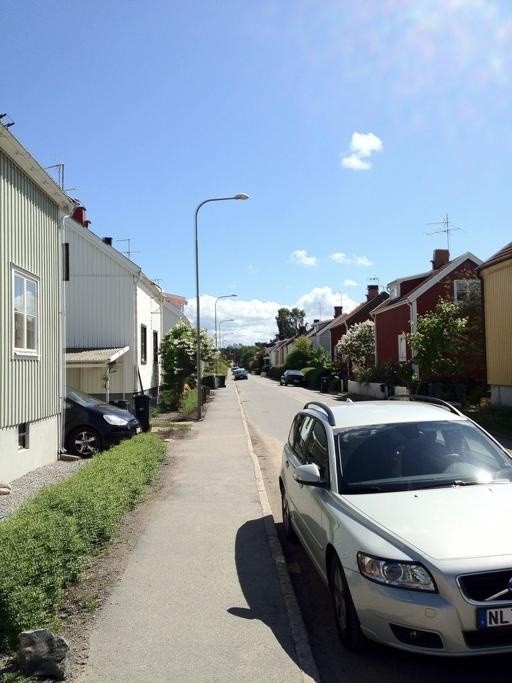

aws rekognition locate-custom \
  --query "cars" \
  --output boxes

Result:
[280,370,305,387]
[279,394,512,658]
[64,385,142,458]
[232,367,248,380]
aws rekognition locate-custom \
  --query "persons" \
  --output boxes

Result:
[429,431,466,470]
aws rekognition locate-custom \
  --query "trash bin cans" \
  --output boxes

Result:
[320,377,329,393]
[134,395,151,433]
[215,375,226,388]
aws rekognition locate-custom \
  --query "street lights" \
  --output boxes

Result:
[196,193,251,419]
[216,293,238,348]
